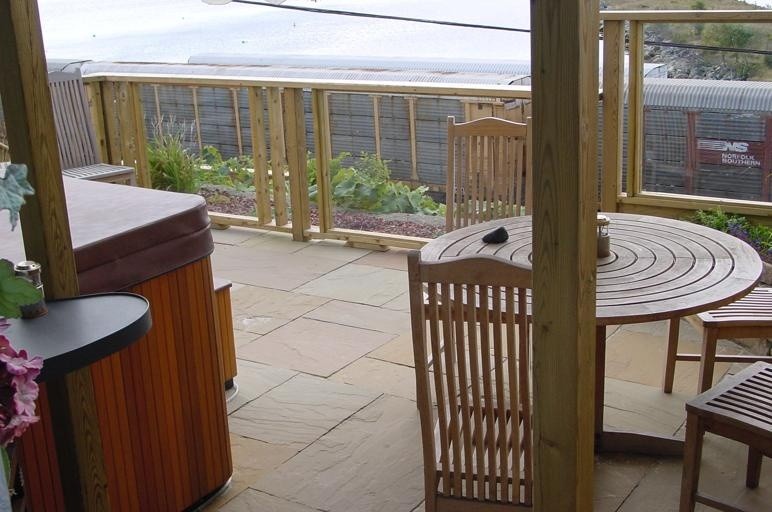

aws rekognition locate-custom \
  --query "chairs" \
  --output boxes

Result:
[677,359,771,512]
[443,111,534,235]
[49,66,138,186]
[659,285,771,396]
[407,248,534,512]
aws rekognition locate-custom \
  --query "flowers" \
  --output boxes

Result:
[0,329,50,459]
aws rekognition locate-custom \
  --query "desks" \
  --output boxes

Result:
[0,290,153,393]
[416,210,766,461]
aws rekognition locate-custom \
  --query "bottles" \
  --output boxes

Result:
[597,215,610,258]
[12,261,49,320]
